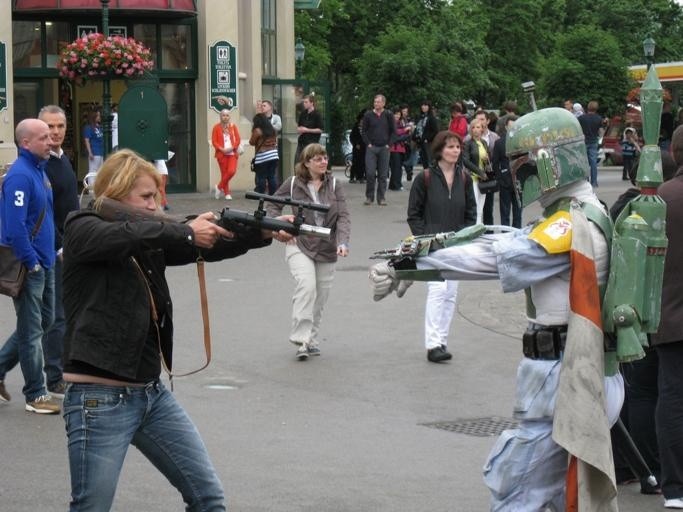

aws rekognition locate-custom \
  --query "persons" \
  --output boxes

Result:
[107,104,118,151]
[82,109,104,193]
[609,149,676,495]
[650,125,683,508]
[265,143,352,358]
[153,160,170,209]
[0,118,61,414]
[407,130,477,362]
[211,108,241,201]
[61,148,297,512]
[38,105,81,393]
[371,107,626,512]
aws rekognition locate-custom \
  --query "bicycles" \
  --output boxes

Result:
[343,158,352,179]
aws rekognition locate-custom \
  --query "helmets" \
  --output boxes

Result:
[506,107,590,208]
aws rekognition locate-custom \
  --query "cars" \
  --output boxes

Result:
[341,129,353,149]
[416,151,422,164]
[317,133,353,166]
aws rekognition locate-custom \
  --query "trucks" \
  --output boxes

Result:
[594,112,644,168]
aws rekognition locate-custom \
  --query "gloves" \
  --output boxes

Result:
[368,256,417,300]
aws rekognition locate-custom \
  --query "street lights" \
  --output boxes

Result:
[642,33,657,73]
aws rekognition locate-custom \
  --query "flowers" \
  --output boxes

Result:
[55,33,157,84]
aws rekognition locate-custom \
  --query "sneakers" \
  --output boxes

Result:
[26,393,60,415]
[297,345,308,358]
[226,193,232,200]
[0,380,11,402]
[428,344,451,362]
[378,198,386,205]
[364,198,372,204]
[46,381,67,399]
[215,184,223,199]
[309,342,321,354]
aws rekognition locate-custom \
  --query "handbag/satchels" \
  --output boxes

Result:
[0,244,26,298]
[480,179,500,194]
[251,158,256,170]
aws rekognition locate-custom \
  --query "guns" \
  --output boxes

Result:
[90,189,331,242]
